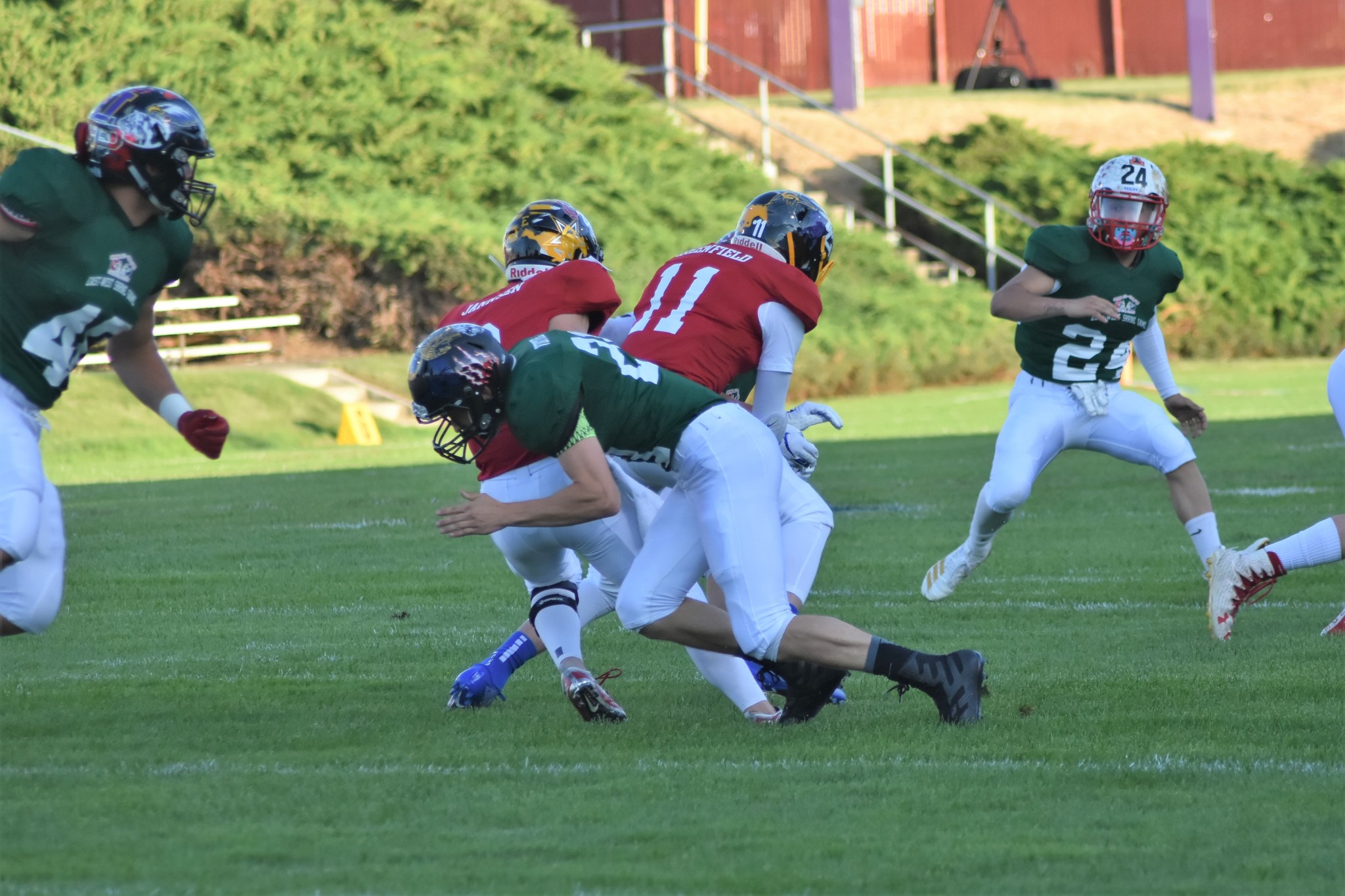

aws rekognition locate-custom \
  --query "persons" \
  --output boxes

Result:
[408,190,986,730]
[1204,350,1345,643]
[921,152,1270,608]
[0,85,230,639]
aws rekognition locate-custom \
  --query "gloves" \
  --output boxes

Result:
[177,409,229,460]
[779,423,818,479]
[785,402,843,431]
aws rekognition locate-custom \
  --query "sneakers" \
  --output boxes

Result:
[746,659,847,705]
[1202,537,1270,583]
[563,667,629,724]
[447,663,505,710]
[745,707,783,725]
[921,539,992,600]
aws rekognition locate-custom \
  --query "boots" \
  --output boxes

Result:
[881,649,987,726]
[753,651,851,725]
[1205,549,1287,643]
[1320,608,1345,636]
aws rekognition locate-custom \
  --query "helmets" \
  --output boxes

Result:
[1086,155,1170,249]
[716,190,834,288]
[74,86,217,227]
[407,323,514,465]
[504,200,604,283]
